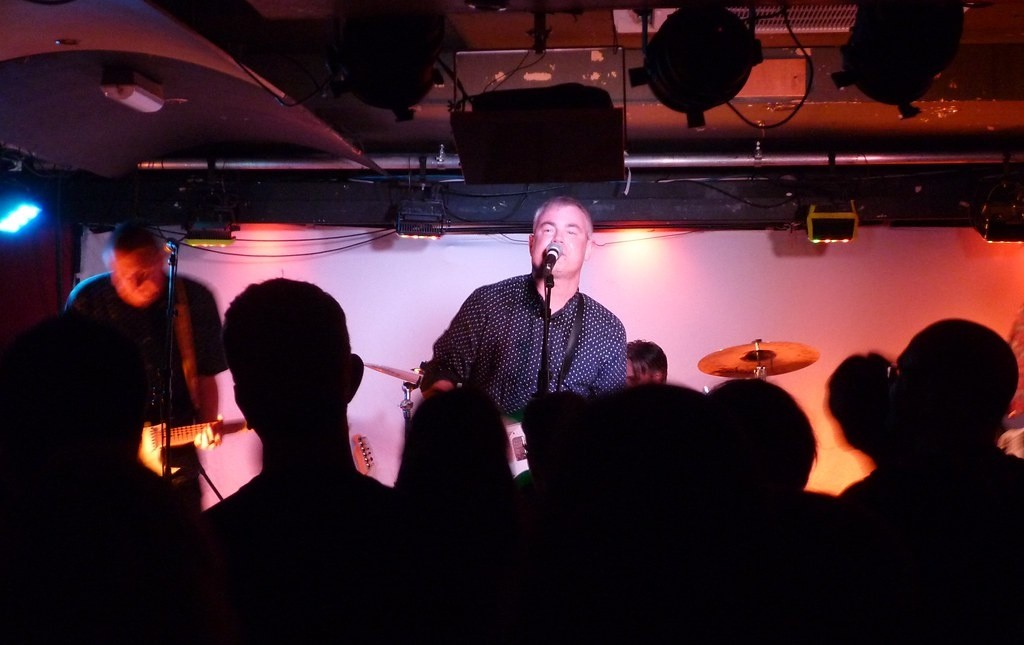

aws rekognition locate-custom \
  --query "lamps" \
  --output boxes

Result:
[627,3,763,132]
[396,199,450,239]
[99,70,165,115]
[973,180,1024,244]
[322,5,447,123]
[806,200,859,243]
[182,224,236,248]
[825,1,965,121]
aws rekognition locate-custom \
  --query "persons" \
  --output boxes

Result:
[627,341,667,387]
[0,223,1024,645]
[421,197,627,404]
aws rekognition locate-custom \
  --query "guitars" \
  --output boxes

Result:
[351,432,376,478]
[137,415,250,475]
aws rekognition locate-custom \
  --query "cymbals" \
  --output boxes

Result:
[363,362,423,387]
[697,340,822,381]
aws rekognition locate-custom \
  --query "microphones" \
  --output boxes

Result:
[545,243,562,270]
[163,238,178,252]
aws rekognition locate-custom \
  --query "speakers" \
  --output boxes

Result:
[450,107,626,186]
